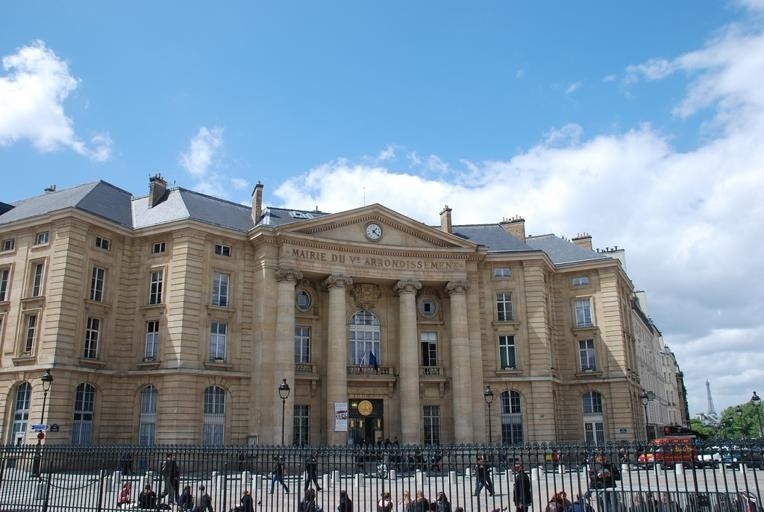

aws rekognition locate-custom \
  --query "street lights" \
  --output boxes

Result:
[482,383,496,467]
[638,387,650,443]
[750,390,764,436]
[30,367,53,479]
[275,377,291,476]
[719,416,735,439]
[735,404,745,438]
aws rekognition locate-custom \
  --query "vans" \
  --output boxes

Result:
[696,444,738,463]
[637,434,700,468]
[721,448,764,471]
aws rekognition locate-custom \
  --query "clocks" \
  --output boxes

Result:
[363,221,383,243]
